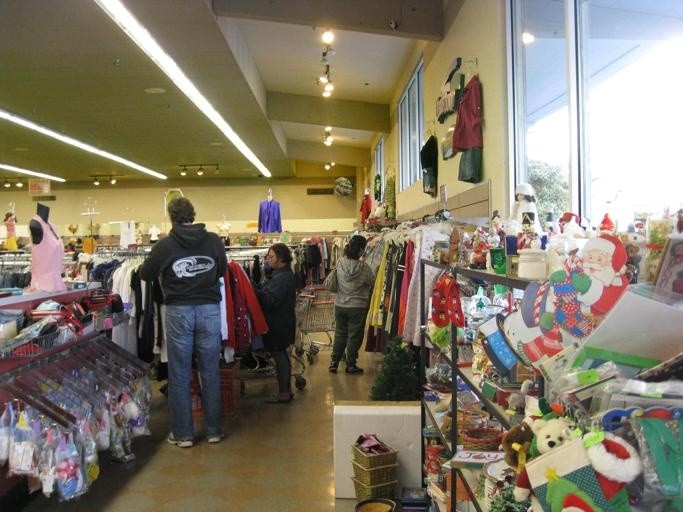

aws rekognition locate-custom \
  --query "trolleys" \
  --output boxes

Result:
[236,293,318,396]
[293,287,335,365]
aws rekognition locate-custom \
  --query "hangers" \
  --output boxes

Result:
[0,255,31,272]
[98,249,152,265]
[363,210,457,236]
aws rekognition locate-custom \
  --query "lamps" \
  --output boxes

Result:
[86,173,121,186]
[0,176,23,189]
[318,29,336,171]
[177,162,223,178]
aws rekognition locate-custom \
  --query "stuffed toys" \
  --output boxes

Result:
[513,418,574,512]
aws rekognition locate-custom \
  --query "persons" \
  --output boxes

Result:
[467,182,581,269]
[3,210,19,250]
[258,196,282,234]
[255,244,298,404]
[23,203,67,294]
[138,197,227,449]
[329,235,375,376]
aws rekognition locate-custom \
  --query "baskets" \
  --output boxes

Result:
[352,460,399,485]
[463,429,502,450]
[352,443,397,469]
[350,478,401,510]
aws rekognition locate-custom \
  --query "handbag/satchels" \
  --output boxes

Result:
[323,270,338,291]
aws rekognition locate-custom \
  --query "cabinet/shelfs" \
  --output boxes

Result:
[418,258,531,512]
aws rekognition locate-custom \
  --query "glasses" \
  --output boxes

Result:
[267,255,274,259]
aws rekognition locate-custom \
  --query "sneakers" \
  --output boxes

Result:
[345,365,363,375]
[208,436,222,443]
[329,364,338,372]
[267,394,293,403]
[167,432,193,447]
